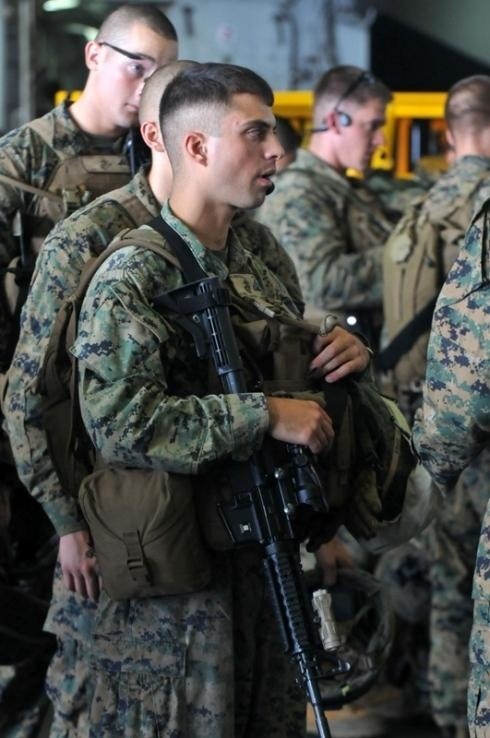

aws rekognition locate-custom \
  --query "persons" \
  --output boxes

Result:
[73,63,375,738]
[2,60,199,738]
[272,118,303,174]
[254,66,400,647]
[365,77,490,737]
[1,3,178,736]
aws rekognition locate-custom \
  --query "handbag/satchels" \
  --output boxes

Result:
[78,467,214,600]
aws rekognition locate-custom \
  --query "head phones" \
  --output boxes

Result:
[312,70,375,134]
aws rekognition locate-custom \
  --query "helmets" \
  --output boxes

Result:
[304,567,395,709]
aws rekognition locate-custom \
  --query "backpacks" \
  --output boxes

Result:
[385,169,490,392]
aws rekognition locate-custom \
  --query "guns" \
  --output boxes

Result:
[156,268,362,738]
